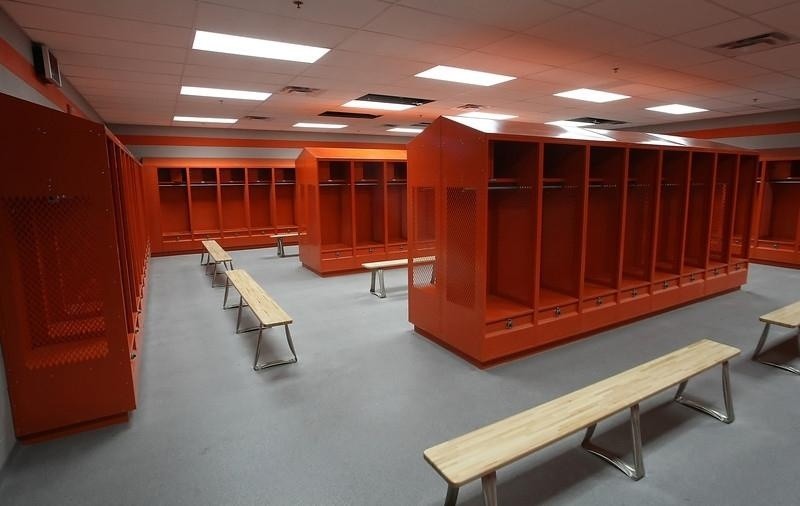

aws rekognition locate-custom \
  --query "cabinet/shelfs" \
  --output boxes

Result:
[407,135,759,368]
[143,166,295,257]
[732,157,799,268]
[294,157,437,277]
[0,127,142,448]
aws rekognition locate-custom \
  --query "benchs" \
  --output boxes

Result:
[200,241,297,371]
[361,256,439,298]
[269,232,298,257]
[421,339,742,506]
[753,299,800,378]
